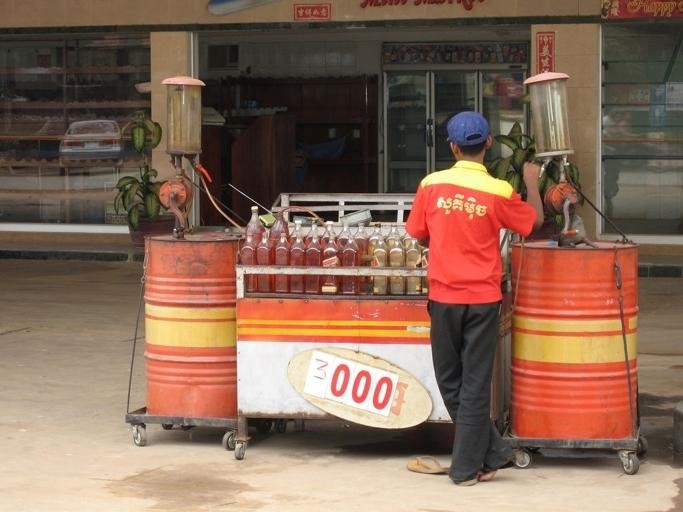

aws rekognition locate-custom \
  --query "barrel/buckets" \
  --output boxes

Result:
[140,229,247,422]
[508,233,642,440]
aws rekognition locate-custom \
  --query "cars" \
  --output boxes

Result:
[58,120,124,176]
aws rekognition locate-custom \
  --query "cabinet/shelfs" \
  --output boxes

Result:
[0,35,151,222]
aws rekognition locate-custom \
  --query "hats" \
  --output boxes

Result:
[446,111,490,146]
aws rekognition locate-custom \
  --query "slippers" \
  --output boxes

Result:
[407,455,450,475]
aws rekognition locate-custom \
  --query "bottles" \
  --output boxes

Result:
[382,43,528,64]
[238,205,429,296]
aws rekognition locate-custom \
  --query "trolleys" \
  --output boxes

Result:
[230,190,523,462]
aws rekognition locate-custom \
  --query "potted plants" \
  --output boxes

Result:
[112,109,176,247]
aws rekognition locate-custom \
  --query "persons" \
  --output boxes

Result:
[405,109,544,487]
[601,109,649,222]
